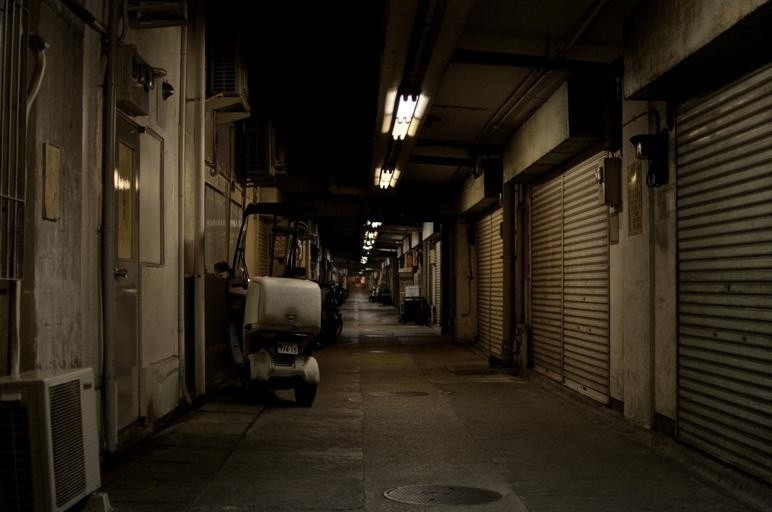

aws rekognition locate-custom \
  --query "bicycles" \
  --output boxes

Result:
[311,281,348,349]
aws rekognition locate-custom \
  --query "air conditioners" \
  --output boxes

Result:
[210,49,250,114]
[1,367,102,512]
